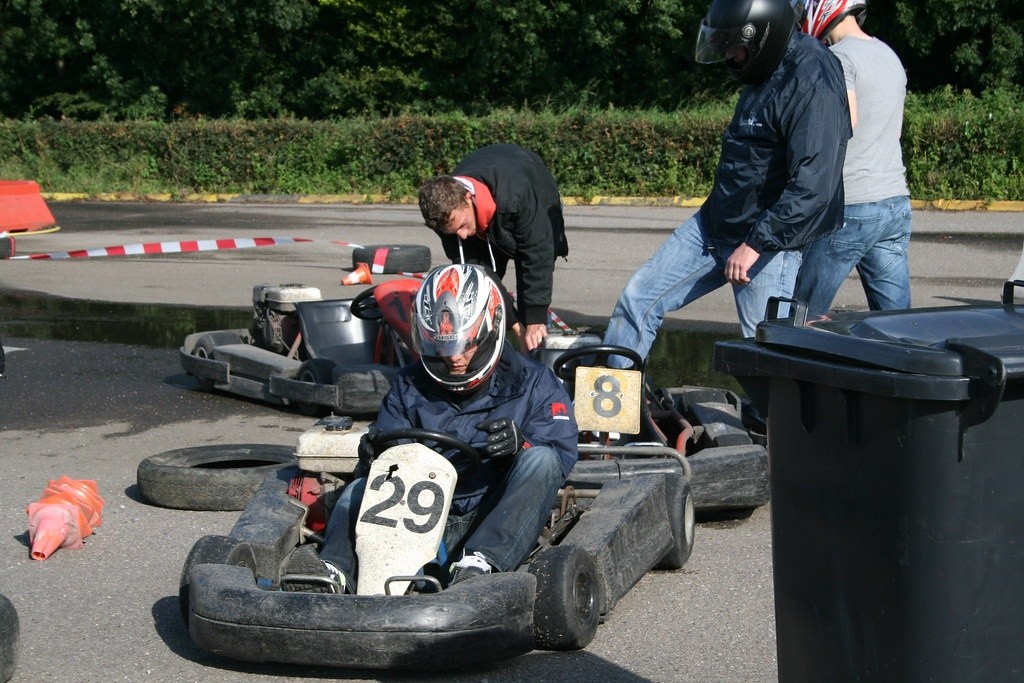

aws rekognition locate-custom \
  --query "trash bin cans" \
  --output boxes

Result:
[712,279,1024,683]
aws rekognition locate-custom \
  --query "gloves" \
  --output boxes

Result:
[357,427,399,468]
[475,418,526,457]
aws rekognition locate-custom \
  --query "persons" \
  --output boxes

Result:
[418,143,569,356]
[789,0,913,322]
[286,264,578,594]
[578,0,854,442]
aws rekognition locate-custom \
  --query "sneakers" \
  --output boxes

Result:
[447,547,492,587]
[286,545,346,594]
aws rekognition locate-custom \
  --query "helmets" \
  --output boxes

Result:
[790,0,866,45]
[705,0,795,84]
[410,264,506,391]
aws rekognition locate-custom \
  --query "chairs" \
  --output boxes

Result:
[530,346,604,403]
[295,299,377,369]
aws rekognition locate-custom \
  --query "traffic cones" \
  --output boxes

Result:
[25,475,106,562]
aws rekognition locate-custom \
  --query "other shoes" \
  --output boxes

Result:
[742,414,767,435]
